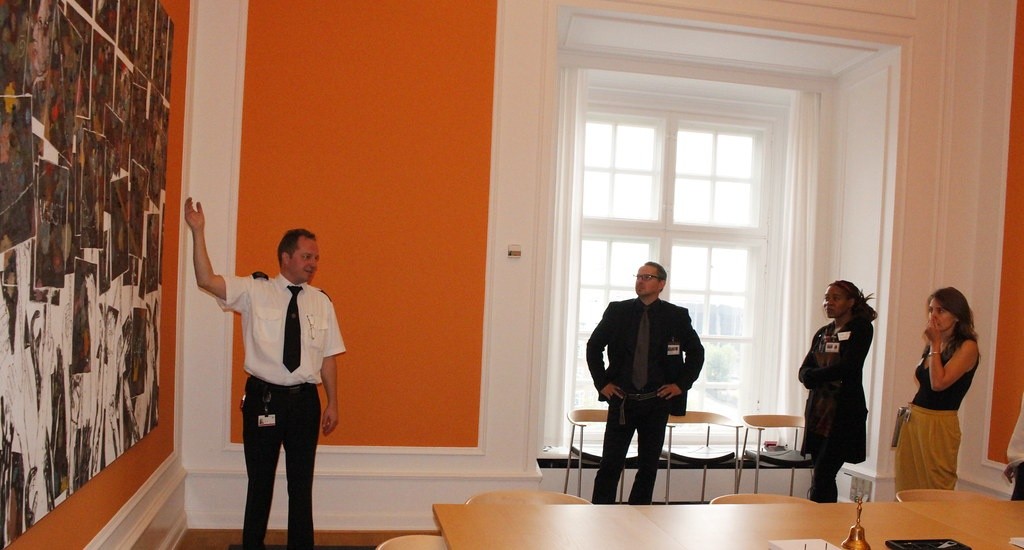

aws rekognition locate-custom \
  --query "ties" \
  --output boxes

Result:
[283,286,303,373]
[632,306,649,390]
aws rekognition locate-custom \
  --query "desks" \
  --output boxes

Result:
[433,500,1024,550]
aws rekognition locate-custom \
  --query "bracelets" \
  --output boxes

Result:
[928,352,941,356]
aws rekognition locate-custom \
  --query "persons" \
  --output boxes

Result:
[184,196,345,549]
[798,280,879,503]
[586,262,705,505]
[891,287,980,501]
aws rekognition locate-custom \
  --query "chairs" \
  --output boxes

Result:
[895,489,998,503]
[564,409,639,503]
[738,415,813,498]
[375,535,448,550]
[464,490,592,505]
[660,412,743,504]
[709,494,818,506]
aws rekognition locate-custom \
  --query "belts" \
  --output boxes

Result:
[626,391,658,401]
[249,375,316,394]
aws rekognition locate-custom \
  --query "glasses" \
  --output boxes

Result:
[637,274,662,281]
